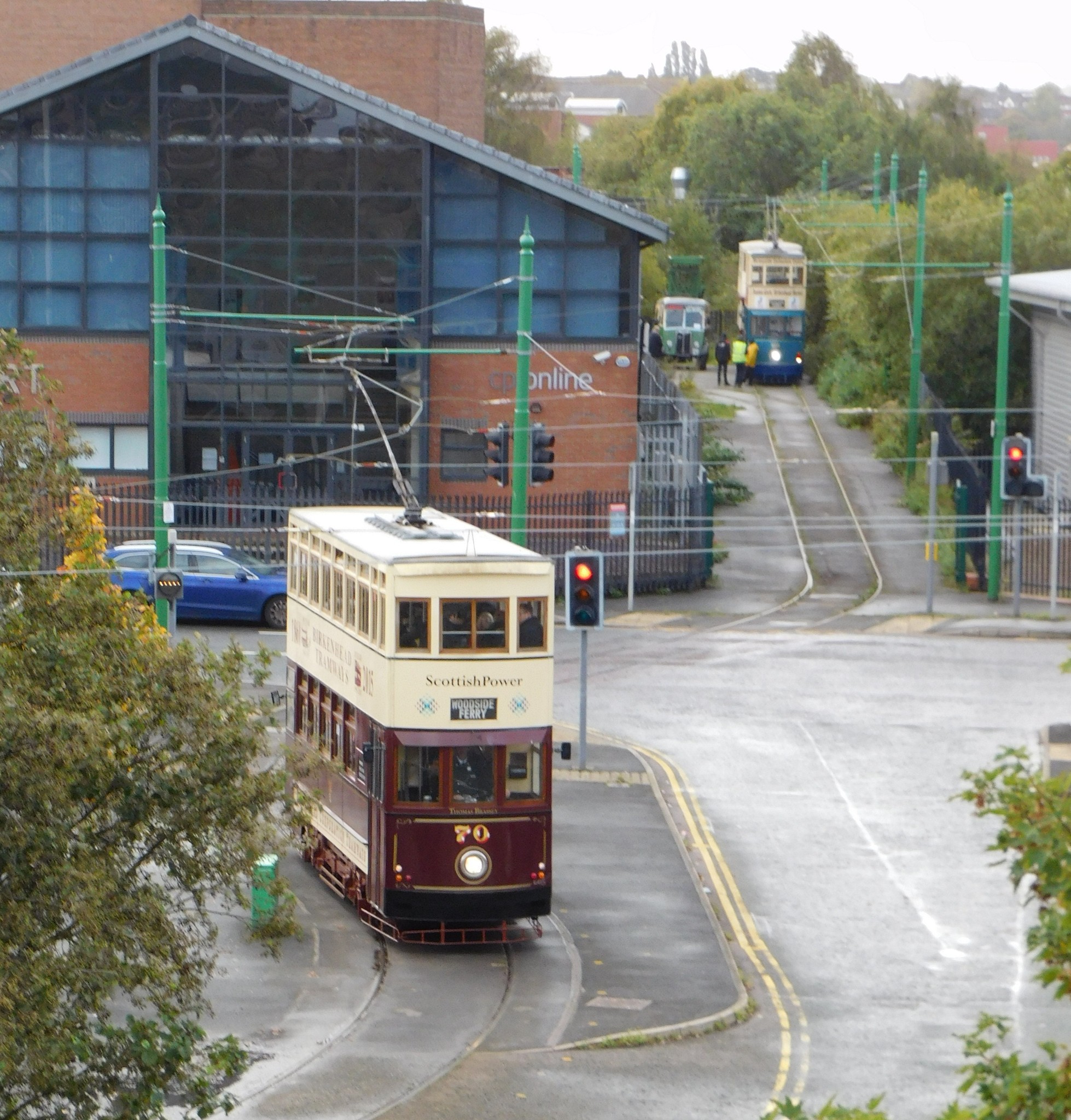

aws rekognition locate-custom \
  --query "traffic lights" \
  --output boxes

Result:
[528,422,556,487]
[565,551,603,630]
[1001,436,1048,499]
[483,421,509,487]
[155,570,183,599]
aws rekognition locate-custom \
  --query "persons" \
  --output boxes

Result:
[649,325,665,368]
[742,336,761,388]
[731,336,748,389]
[714,333,734,387]
[393,740,498,804]
[395,596,547,656]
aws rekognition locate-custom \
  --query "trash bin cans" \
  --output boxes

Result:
[250,852,280,930]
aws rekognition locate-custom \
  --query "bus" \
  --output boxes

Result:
[739,195,806,385]
[282,370,555,948]
[655,297,706,359]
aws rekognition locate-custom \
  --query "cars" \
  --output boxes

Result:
[100,540,287,630]
